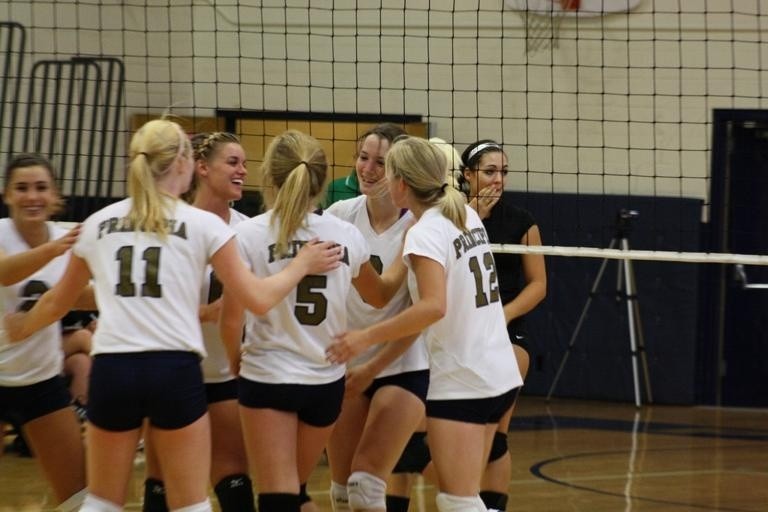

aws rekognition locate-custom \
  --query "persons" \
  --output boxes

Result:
[1,118,549,511]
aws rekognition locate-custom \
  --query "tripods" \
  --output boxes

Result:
[545,210,653,409]
